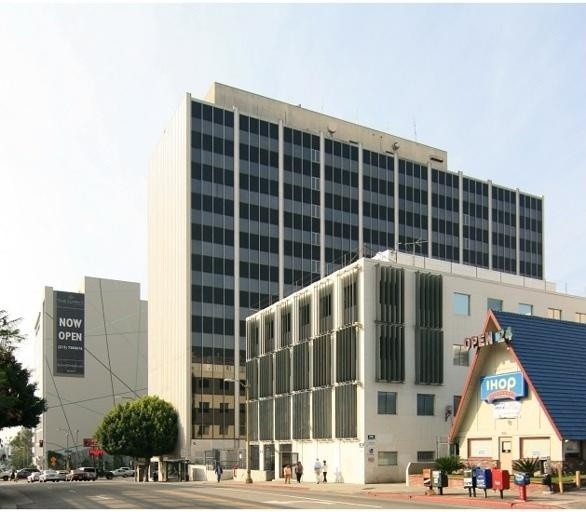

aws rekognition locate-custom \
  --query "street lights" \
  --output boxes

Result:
[57,427,80,468]
[222,377,252,483]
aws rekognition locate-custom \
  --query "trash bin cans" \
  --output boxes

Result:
[540,460,548,474]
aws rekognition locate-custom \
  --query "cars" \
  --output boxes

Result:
[0,467,135,482]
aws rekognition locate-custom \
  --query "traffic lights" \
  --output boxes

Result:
[39,440,44,447]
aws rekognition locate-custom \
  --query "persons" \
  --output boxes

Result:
[314,458,321,484]
[215,464,223,482]
[295,462,303,483]
[283,464,292,484]
[321,460,328,483]
